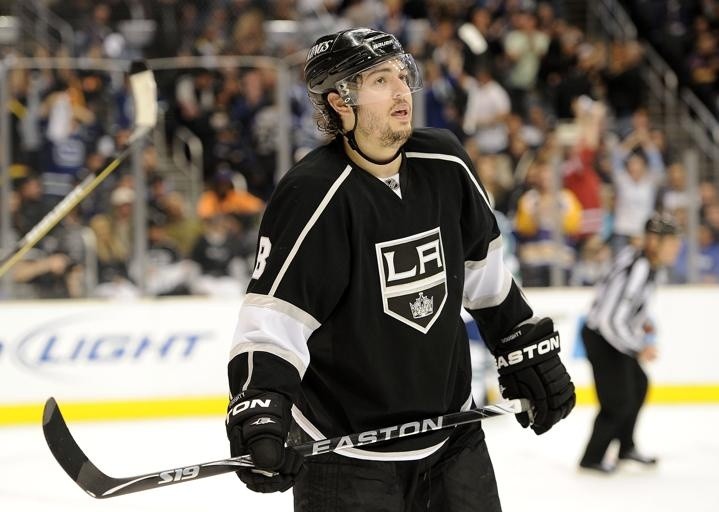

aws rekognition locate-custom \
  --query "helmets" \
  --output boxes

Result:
[303,27,405,95]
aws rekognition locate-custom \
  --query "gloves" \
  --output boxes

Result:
[225,391,307,493]
[491,316,576,436]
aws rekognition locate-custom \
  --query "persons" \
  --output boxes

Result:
[372,0,718,288]
[222,24,576,511]
[574,211,688,481]
[2,1,383,293]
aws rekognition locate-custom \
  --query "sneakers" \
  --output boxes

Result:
[623,446,658,465]
[580,456,616,473]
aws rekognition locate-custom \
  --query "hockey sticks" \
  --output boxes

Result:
[43,396,530,499]
[0,58,158,275]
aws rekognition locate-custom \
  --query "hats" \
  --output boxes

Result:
[644,216,682,235]
